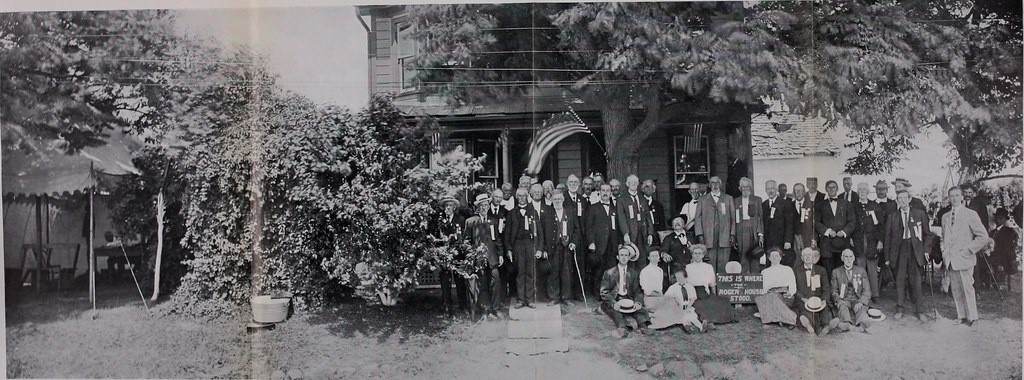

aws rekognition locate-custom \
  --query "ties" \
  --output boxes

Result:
[573,196,591,202]
[845,192,849,202]
[446,208,501,223]
[768,200,802,216]
[631,194,650,219]
[994,230,999,241]
[623,268,626,292]
[902,200,971,240]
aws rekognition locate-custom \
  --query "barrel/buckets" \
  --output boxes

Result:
[249,294,291,324]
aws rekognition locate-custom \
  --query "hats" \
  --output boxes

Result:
[873,177,913,188]
[617,241,639,262]
[804,236,888,321]
[992,208,1011,220]
[539,258,551,276]
[960,180,978,192]
[612,299,641,314]
[667,213,687,228]
[437,194,493,207]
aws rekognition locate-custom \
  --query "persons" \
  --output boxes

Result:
[464,170,665,321]
[662,269,709,335]
[973,207,1019,290]
[101,230,127,285]
[939,186,989,327]
[791,247,840,339]
[658,212,699,285]
[830,248,873,334]
[948,182,990,304]
[724,147,749,198]
[683,244,740,331]
[753,246,797,330]
[431,195,472,319]
[638,246,687,329]
[681,174,952,302]
[883,190,933,321]
[600,247,658,338]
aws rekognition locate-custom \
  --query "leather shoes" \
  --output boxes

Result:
[612,315,866,338]
[873,294,978,327]
[446,297,601,323]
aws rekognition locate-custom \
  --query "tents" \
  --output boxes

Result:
[1,117,170,312]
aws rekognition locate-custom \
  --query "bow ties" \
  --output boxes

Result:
[601,202,610,206]
[689,195,720,203]
[679,233,685,237]
[518,205,529,210]
[830,198,837,202]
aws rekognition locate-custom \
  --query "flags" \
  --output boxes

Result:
[520,106,592,177]
